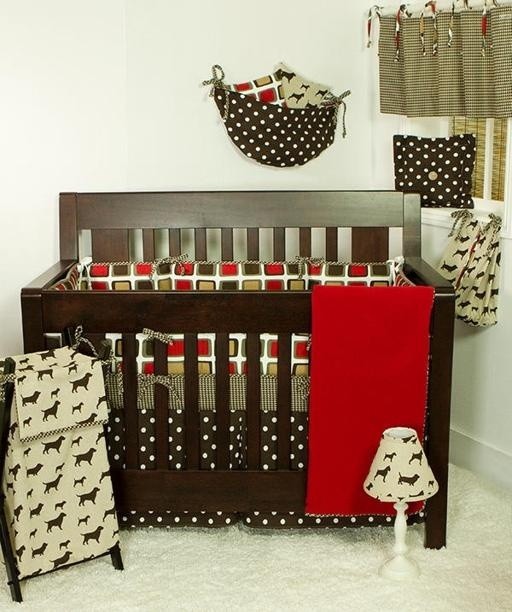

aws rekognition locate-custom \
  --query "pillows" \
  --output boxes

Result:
[394,132,477,209]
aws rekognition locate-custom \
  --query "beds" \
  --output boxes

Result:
[20,190,455,548]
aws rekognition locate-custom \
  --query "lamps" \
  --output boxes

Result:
[363,424,439,581]
